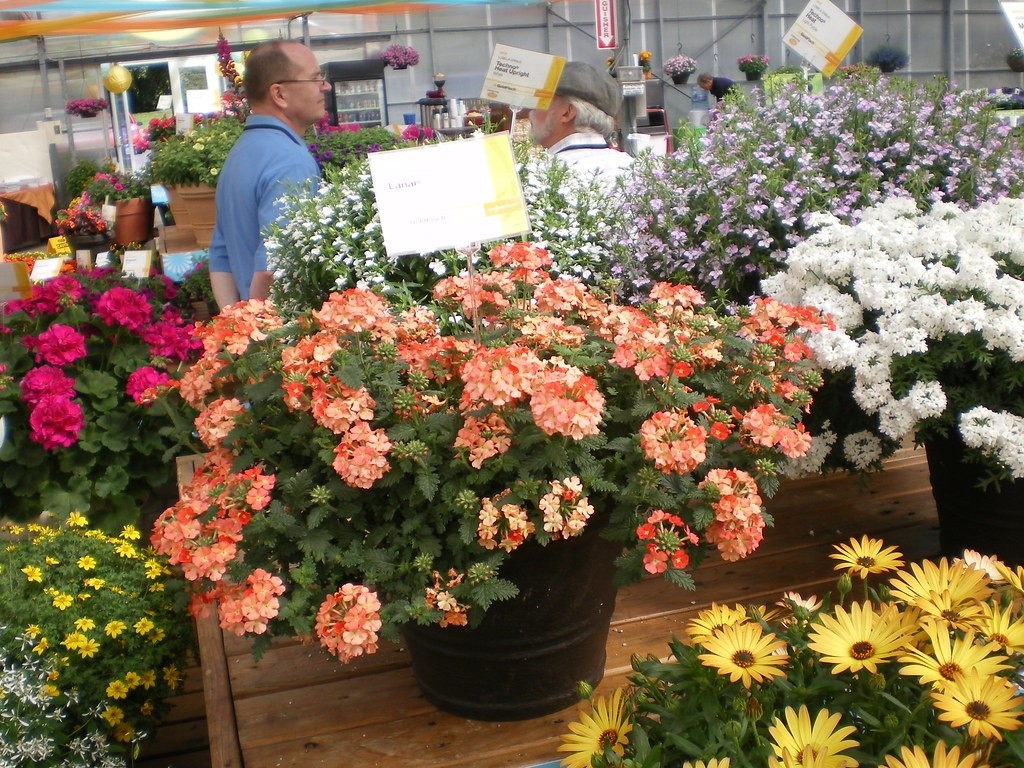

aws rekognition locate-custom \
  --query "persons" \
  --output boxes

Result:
[209,42,332,310]
[527,62,634,198]
[698,73,740,106]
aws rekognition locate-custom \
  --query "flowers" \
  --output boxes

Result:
[0,27,1024,768]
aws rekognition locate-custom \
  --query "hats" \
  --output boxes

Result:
[557,61,622,117]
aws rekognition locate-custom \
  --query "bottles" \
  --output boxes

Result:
[441,108,449,128]
[335,83,380,123]
[433,109,442,129]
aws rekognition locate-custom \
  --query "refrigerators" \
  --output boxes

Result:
[318,58,388,133]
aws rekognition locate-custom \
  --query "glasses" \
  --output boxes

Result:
[269,69,327,87]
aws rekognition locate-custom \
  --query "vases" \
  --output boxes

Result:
[397,63,407,69]
[176,182,216,246]
[609,70,616,78]
[924,442,1024,574]
[398,513,624,721]
[113,198,149,244]
[671,73,690,84]
[81,112,96,117]
[67,234,109,259]
[1007,60,1024,72]
[644,71,652,79]
[165,184,191,226]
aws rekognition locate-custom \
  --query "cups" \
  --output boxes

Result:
[448,98,466,127]
